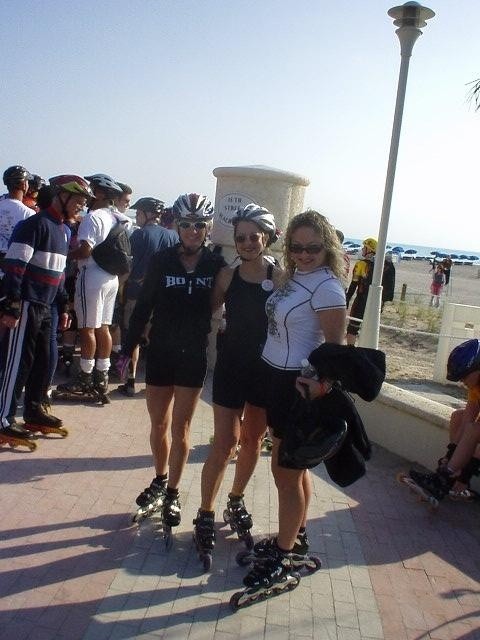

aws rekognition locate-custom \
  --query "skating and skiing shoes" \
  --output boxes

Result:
[438,445,476,503]
[223,494,255,550]
[22,401,69,437]
[396,467,456,511]
[160,494,181,553]
[0,424,38,452]
[95,369,110,403]
[236,532,321,571]
[51,370,94,402]
[193,508,216,571]
[133,478,166,523]
[229,546,301,611]
[63,349,75,373]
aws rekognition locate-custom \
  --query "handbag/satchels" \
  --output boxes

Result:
[275,384,349,468]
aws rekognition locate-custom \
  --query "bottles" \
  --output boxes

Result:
[301,357,320,382]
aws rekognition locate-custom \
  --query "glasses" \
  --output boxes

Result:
[235,232,261,243]
[287,245,323,254]
[179,221,207,230]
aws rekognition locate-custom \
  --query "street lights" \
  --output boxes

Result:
[356,1,436,366]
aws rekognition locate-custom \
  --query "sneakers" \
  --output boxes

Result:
[117,383,136,396]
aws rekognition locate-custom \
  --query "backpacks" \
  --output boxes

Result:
[91,214,131,275]
[360,258,395,301]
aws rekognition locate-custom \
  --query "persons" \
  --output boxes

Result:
[409,337,480,501]
[430,265,444,308]
[439,254,452,285]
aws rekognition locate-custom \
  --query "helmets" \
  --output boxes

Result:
[446,338,480,381]
[84,174,124,194]
[231,203,276,233]
[3,165,47,186]
[129,197,165,213]
[49,175,96,200]
[362,237,379,254]
[172,192,215,219]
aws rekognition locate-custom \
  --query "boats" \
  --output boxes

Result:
[342,239,479,266]
